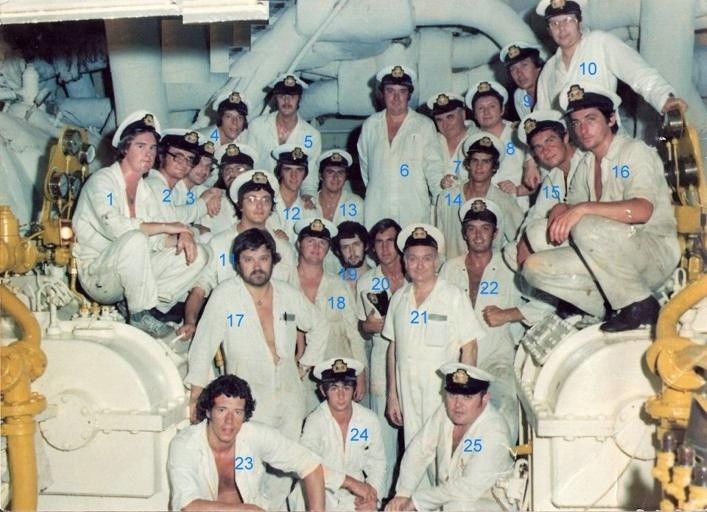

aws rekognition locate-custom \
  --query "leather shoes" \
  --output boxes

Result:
[600,293,663,336]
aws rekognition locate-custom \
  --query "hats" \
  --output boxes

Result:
[374,39,546,118]
[437,363,494,392]
[225,168,282,210]
[110,108,354,166]
[455,198,504,226]
[516,82,621,148]
[360,291,388,324]
[531,1,594,39]
[461,134,507,156]
[394,222,445,251]
[290,219,338,243]
[309,358,366,382]
[208,72,311,123]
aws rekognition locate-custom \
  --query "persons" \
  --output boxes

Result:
[71,0,688,511]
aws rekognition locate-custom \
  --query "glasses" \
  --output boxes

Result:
[542,15,578,29]
[237,193,274,206]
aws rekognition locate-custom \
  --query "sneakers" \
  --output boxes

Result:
[111,296,183,341]
